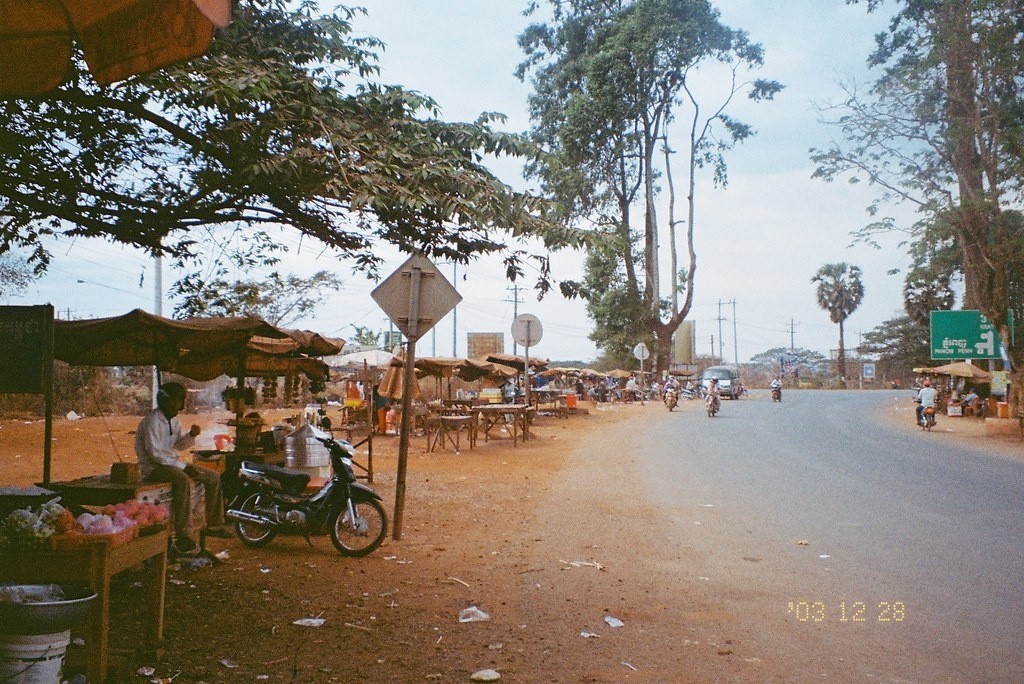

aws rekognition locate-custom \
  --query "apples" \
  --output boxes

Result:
[101,499,169,527]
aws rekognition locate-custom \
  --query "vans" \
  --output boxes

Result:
[699,365,741,400]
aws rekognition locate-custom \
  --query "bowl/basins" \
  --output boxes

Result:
[0,584,98,634]
[345,400,362,407]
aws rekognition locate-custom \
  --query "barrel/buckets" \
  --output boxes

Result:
[0,629,72,684]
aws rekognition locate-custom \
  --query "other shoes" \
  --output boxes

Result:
[176,536,196,551]
[206,528,235,538]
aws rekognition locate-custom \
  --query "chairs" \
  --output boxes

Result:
[966,398,979,417]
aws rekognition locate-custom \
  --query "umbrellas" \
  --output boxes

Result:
[377,347,420,436]
[390,353,549,416]
[0,0,231,98]
[330,350,396,437]
[543,367,632,401]
[669,370,694,380]
[51,309,345,453]
[912,362,993,391]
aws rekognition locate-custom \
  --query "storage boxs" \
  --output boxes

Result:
[996,402,1007,418]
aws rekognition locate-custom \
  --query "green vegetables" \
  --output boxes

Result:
[0,503,65,554]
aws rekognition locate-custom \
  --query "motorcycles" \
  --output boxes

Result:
[770,385,781,402]
[911,396,941,433]
[662,387,680,411]
[225,415,390,558]
[704,390,721,418]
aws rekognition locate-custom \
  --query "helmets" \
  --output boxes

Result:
[924,381,931,386]
[157,382,186,413]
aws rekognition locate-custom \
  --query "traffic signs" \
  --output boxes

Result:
[930,308,1014,361]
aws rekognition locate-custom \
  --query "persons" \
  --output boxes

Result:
[770,375,782,394]
[916,381,937,425]
[705,376,723,404]
[135,382,234,555]
[951,388,979,414]
[588,377,608,403]
[663,379,678,401]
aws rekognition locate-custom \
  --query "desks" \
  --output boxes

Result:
[426,416,472,453]
[473,405,525,447]
[52,473,223,573]
[0,526,170,681]
[531,389,559,413]
